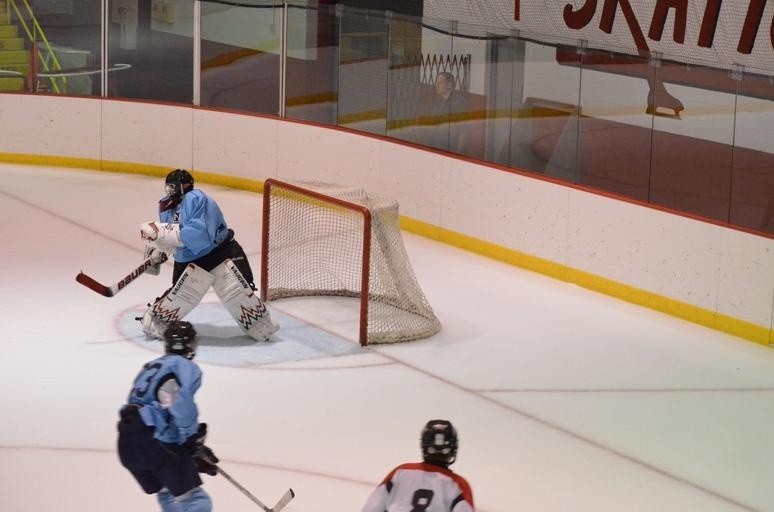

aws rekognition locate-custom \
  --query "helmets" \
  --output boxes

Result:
[164,168,194,201]
[163,320,198,360]
[419,418,458,466]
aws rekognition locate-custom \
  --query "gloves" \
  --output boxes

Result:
[192,442,218,476]
[141,220,185,250]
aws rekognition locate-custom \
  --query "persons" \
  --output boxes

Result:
[117,319,221,512]
[135,168,282,345]
[427,70,473,157]
[358,417,478,512]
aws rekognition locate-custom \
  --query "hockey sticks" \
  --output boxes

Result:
[75,258,153,297]
[198,453,295,512]
